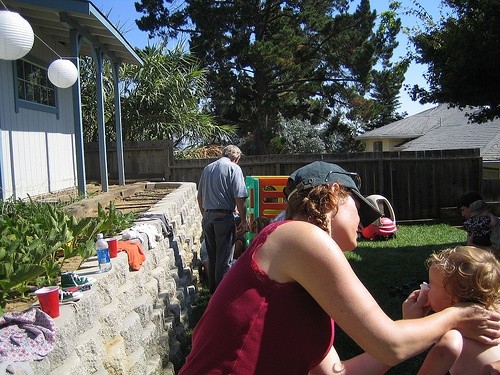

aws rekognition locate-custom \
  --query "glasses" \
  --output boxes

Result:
[324,171,361,191]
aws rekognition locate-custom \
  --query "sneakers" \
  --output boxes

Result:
[61,272,96,290]
[58,289,83,307]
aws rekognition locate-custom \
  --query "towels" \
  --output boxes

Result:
[0,306,57,364]
[116,240,147,271]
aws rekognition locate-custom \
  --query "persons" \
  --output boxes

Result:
[199,212,271,279]
[177,160,500,375]
[458,192,500,251]
[416,245,500,375]
[197,144,248,297]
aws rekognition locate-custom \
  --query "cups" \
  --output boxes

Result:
[96,234,112,273]
[34,286,60,317]
[105,238,117,258]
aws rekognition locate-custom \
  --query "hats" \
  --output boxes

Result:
[456,215,492,237]
[465,200,489,218]
[283,161,385,229]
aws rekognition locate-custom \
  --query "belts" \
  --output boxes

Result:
[204,209,233,214]
